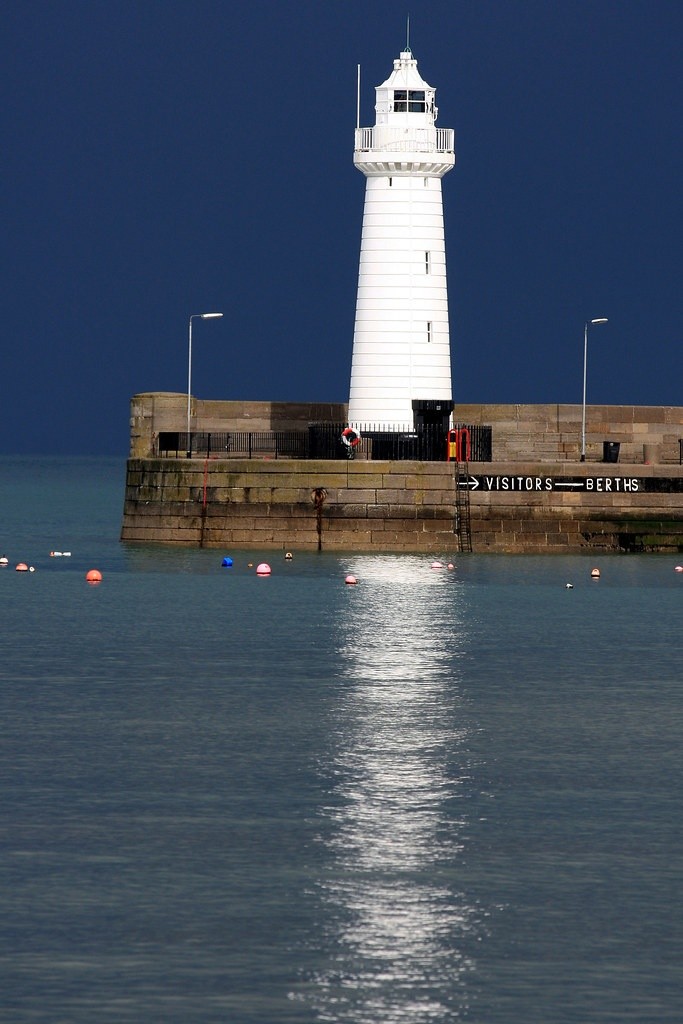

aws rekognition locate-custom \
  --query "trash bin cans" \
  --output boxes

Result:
[678,439,683,465]
[602,441,620,462]
[643,443,659,465]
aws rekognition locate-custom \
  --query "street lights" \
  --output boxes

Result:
[580,317,610,461]
[186,313,225,459]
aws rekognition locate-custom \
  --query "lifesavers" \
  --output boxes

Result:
[339,425,362,447]
